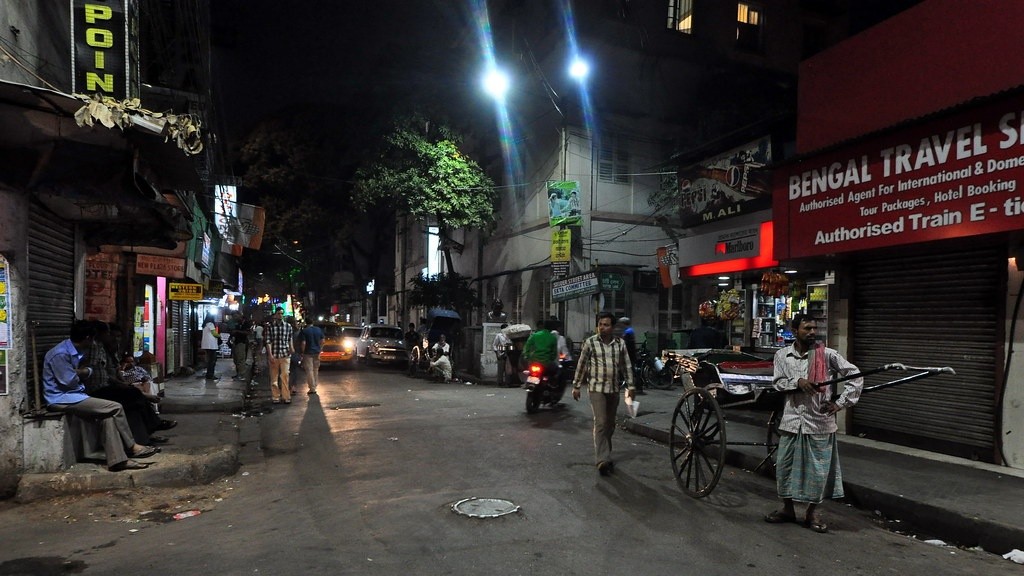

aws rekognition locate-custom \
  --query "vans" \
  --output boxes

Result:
[357,323,409,368]
[302,321,354,369]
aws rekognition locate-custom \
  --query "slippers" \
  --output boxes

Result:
[803,513,828,533]
[128,445,158,459]
[111,461,149,471]
[763,510,797,524]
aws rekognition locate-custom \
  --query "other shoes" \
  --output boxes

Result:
[150,419,177,431]
[272,399,280,404]
[232,374,246,381]
[142,435,169,446]
[599,461,615,477]
[209,376,218,380]
[283,398,291,404]
[290,387,297,394]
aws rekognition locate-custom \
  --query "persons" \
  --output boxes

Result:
[487,298,508,323]
[765,313,862,533]
[492,324,515,386]
[264,306,295,403]
[568,190,582,216]
[618,316,639,387]
[299,317,322,394]
[571,311,636,474]
[405,323,420,376]
[42,319,178,471]
[427,333,453,381]
[200,310,263,380]
[521,320,573,393]
[547,193,562,217]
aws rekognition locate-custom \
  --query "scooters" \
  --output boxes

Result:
[522,354,571,415]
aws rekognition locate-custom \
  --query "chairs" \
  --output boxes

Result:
[688,352,776,402]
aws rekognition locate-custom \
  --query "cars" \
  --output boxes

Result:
[334,321,365,355]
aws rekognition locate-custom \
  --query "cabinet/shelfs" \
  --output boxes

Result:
[756,287,777,346]
[807,284,832,348]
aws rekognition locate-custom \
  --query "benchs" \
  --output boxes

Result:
[23,410,103,473]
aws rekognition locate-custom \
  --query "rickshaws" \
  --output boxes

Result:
[668,360,956,502]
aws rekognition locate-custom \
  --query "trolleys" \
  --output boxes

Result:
[408,346,438,378]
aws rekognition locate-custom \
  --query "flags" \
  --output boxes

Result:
[227,203,265,250]
[220,220,243,256]
